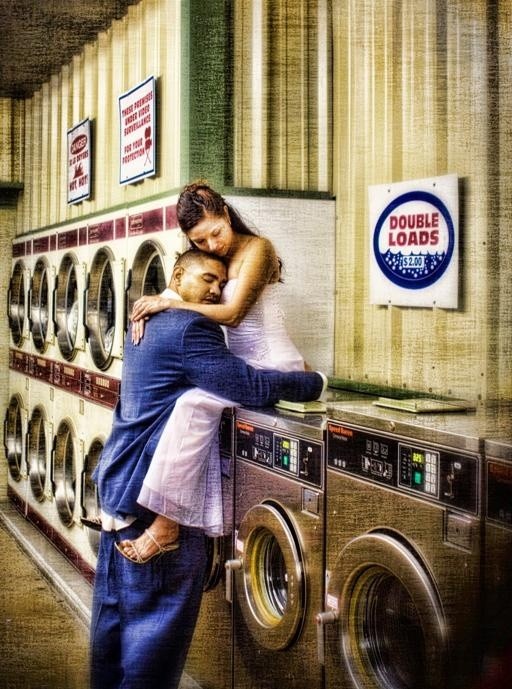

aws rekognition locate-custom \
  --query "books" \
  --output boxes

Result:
[370,395,475,415]
[273,399,335,415]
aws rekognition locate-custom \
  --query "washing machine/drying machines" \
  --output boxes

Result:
[1,181,324,688]
[324,394,511,686]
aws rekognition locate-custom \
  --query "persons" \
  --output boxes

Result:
[81,247,327,688]
[78,179,309,566]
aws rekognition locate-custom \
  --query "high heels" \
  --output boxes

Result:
[113,529,179,565]
